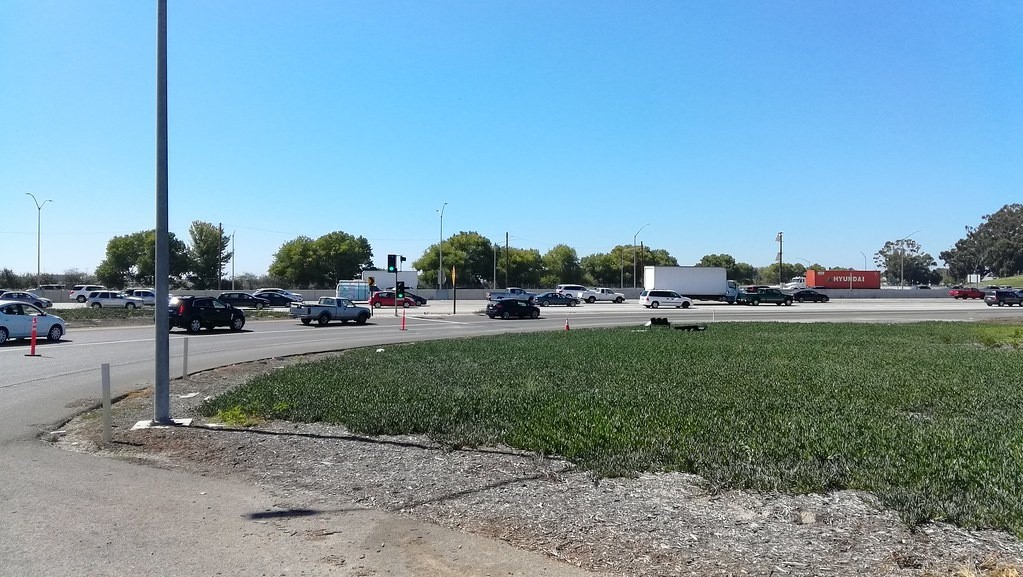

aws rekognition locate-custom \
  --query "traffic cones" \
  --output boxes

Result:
[563,318,570,331]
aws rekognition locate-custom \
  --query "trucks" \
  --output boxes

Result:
[643,265,742,305]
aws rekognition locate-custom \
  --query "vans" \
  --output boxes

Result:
[336,281,383,300]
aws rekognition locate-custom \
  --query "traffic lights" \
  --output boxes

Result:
[400,256,408,263]
[387,254,396,274]
[396,281,405,298]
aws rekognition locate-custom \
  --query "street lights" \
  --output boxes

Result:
[900,231,921,297]
[25,192,53,291]
[800,257,811,268]
[857,248,866,270]
[436,202,448,290]
[633,223,650,291]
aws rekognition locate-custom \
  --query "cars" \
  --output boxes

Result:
[789,289,829,302]
[532,292,579,307]
[0,300,66,344]
[250,288,304,308]
[368,290,416,308]
[404,291,427,306]
[0,287,54,308]
[948,285,963,289]
[216,291,271,310]
[486,298,541,319]
[981,284,1015,291]
[916,285,931,289]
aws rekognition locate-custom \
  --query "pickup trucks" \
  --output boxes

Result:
[485,287,539,302]
[581,287,625,303]
[290,296,371,326]
[736,285,793,306]
[948,287,986,299]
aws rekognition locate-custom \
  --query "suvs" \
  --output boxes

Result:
[983,289,1023,307]
[790,276,806,287]
[122,288,172,304]
[152,293,246,334]
[25,284,66,292]
[639,290,692,308]
[86,290,144,311]
[69,284,108,303]
[556,283,589,299]
